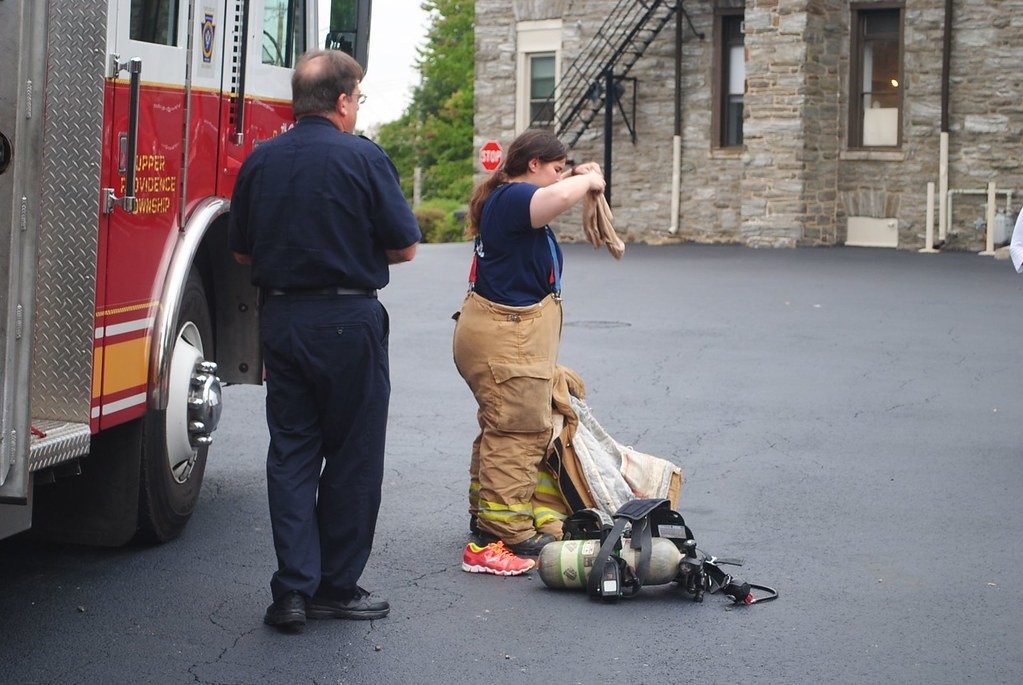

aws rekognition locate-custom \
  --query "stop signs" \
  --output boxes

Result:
[480,141,503,172]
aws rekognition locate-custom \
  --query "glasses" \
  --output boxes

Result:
[348,93,367,104]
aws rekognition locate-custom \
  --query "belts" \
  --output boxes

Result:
[262,284,377,296]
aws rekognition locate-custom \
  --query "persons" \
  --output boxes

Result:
[453,130,605,557]
[229,49,421,630]
[1009,207,1023,273]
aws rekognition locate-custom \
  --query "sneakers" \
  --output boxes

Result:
[305,584,390,620]
[478,532,556,555]
[470,514,490,534]
[462,541,535,575]
[263,589,307,625]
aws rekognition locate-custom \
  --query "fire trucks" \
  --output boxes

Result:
[1,0,372,552]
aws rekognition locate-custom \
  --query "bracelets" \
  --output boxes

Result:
[571,166,577,175]
